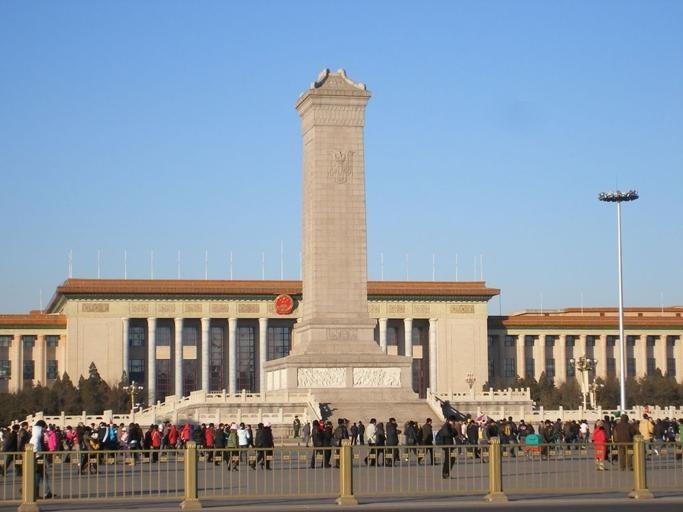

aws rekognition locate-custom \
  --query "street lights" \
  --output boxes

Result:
[570,352,598,410]
[465,369,477,390]
[588,379,604,410]
[599,190,639,415]
[123,381,143,409]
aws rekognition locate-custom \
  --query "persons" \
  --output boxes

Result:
[293,413,683,479]
[0,419,273,498]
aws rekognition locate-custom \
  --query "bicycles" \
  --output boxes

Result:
[605,447,618,465]
